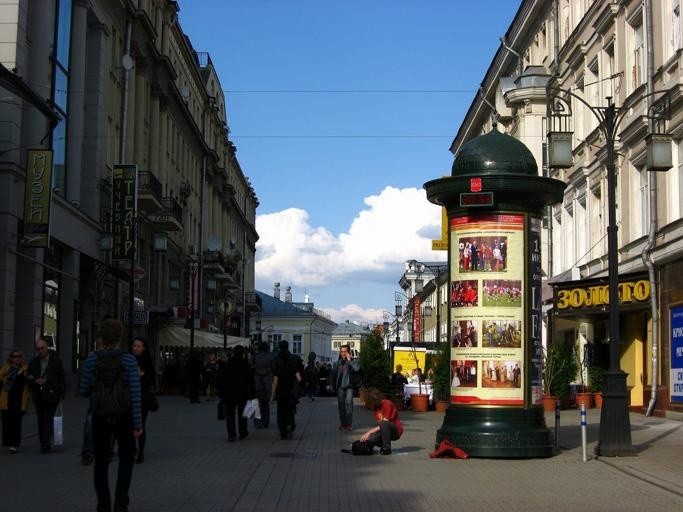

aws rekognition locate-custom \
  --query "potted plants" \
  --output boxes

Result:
[409,346,429,412]
[592,368,603,409]
[430,342,449,411]
[358,331,404,412]
[572,344,592,409]
[542,345,565,411]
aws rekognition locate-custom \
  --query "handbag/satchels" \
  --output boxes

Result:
[352,440,370,455]
[216,400,226,420]
[147,393,158,412]
[53,415,64,446]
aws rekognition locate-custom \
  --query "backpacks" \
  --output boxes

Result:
[92,350,129,414]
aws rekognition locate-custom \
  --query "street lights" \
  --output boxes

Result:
[166,253,263,349]
[383,259,441,351]
[542,83,673,462]
[97,205,170,357]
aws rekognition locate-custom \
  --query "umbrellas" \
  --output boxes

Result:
[156,325,251,352]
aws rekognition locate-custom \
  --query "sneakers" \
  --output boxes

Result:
[380,449,391,455]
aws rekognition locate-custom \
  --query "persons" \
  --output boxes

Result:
[360,388,403,455]
[391,365,434,398]
[485,321,515,345]
[459,239,506,271]
[332,345,360,431]
[202,353,215,402]
[0,351,32,455]
[453,283,477,306]
[217,344,257,441]
[130,337,160,464]
[313,362,333,397]
[79,320,143,512]
[271,340,302,440]
[27,339,67,454]
[452,363,477,387]
[453,325,478,346]
[484,283,522,304]
[486,364,520,387]
